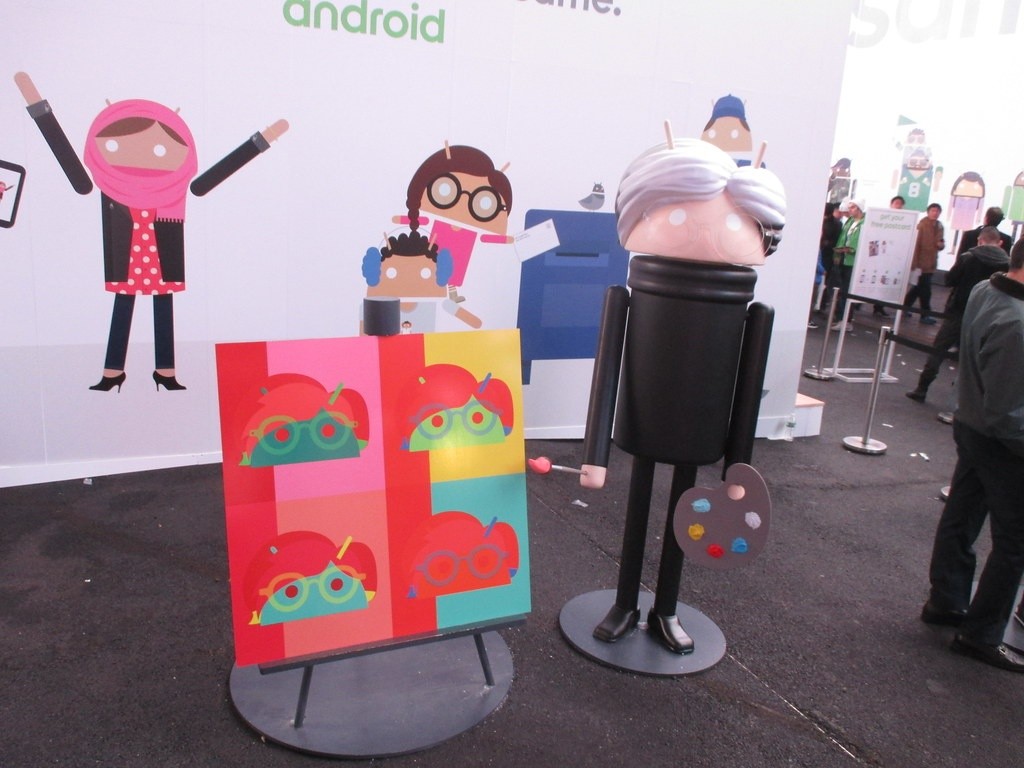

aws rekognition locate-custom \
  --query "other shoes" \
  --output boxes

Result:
[851,309,857,321]
[904,305,912,316]
[948,346,958,353]
[813,312,825,328]
[874,304,889,316]
[920,308,936,324]
[808,320,819,329]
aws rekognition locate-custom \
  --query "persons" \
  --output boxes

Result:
[831,199,865,332]
[919,242,1024,671]
[857,197,905,315]
[807,253,824,329]
[820,203,842,271]
[907,225,1011,404]
[902,203,945,324]
[581,139,786,653]
[956,207,1012,259]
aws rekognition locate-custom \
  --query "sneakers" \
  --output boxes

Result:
[831,320,854,331]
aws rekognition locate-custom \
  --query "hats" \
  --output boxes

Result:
[849,197,865,212]
[839,197,851,212]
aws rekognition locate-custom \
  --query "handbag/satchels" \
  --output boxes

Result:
[909,268,922,286]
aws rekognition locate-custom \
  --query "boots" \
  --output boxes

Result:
[905,374,933,403]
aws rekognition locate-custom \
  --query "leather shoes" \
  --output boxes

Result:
[922,597,969,627]
[956,632,1024,672]
